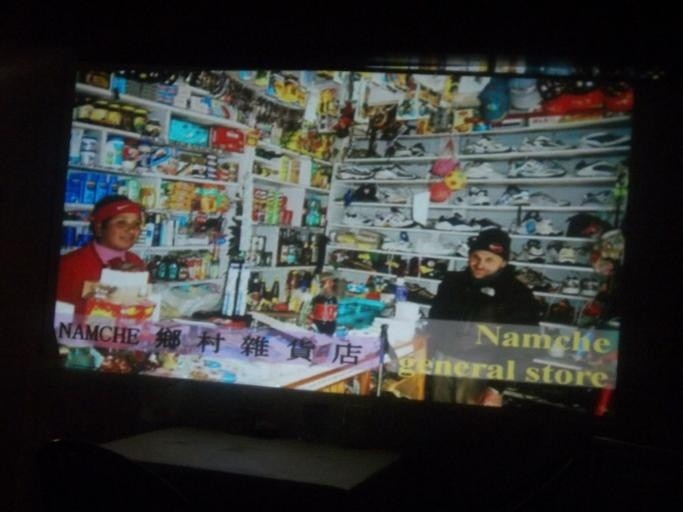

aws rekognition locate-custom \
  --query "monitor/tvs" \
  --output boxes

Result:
[31,42,668,461]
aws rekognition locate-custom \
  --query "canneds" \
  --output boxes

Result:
[73,100,162,137]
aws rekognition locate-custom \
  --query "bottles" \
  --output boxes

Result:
[247,271,280,311]
[279,228,320,268]
[63,170,117,204]
[313,280,338,337]
[149,251,191,282]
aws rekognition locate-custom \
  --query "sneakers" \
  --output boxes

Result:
[327,129,631,326]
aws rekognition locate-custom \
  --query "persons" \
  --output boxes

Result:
[53,194,153,376]
[426,228,539,409]
